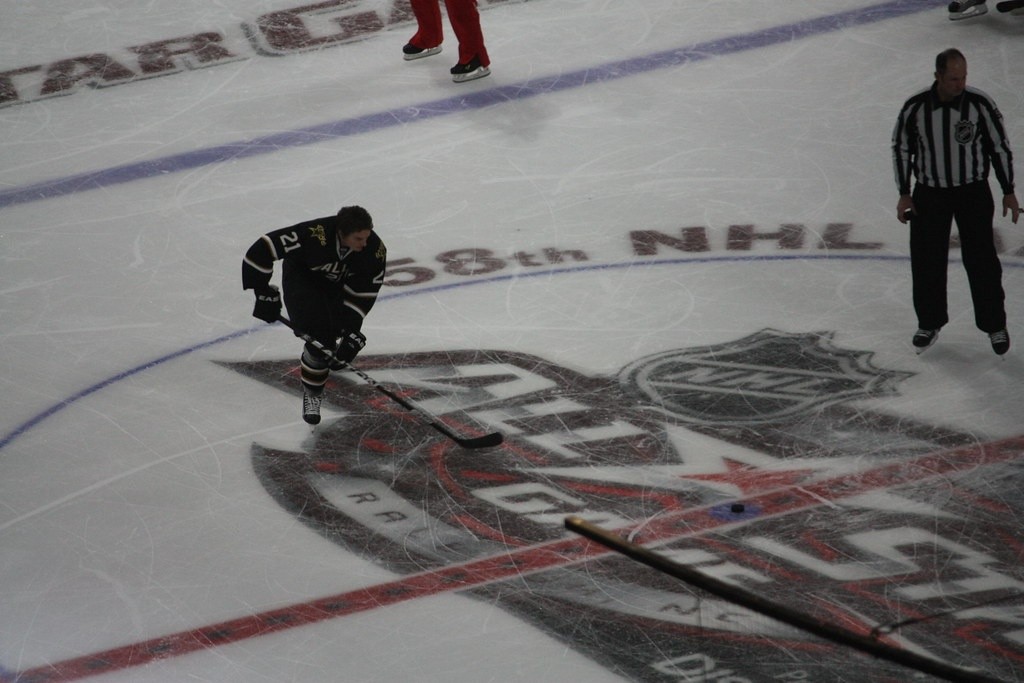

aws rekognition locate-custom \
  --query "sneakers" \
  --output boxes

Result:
[949,0,985,12]
[303,385,322,425]
[997,0,1024,13]
[403,43,424,54]
[450,55,482,75]
[912,328,941,347]
[989,326,1010,355]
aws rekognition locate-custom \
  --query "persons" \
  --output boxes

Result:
[403,0,491,82]
[891,49,1019,360]
[242,205,387,433]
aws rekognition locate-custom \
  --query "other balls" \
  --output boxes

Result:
[731,505,744,512]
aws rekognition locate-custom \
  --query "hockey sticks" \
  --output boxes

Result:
[274,314,504,450]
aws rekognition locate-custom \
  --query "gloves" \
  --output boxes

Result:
[253,281,282,323]
[323,327,366,371]
